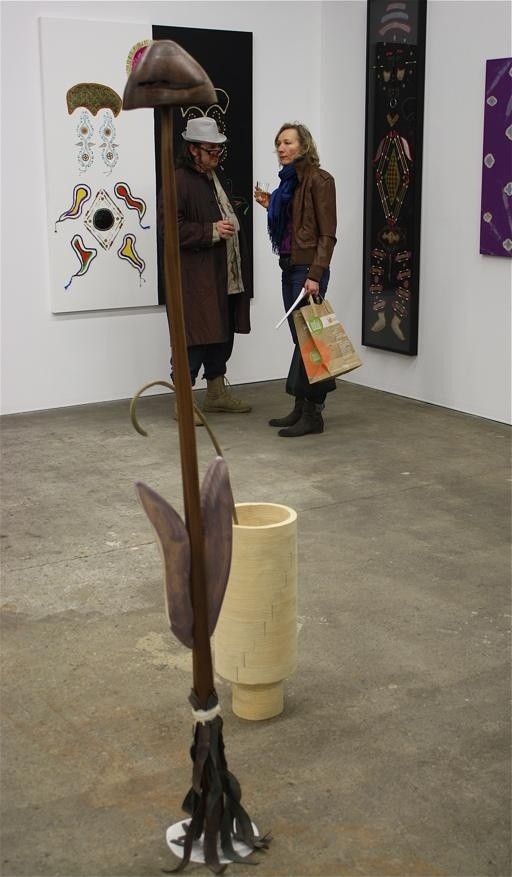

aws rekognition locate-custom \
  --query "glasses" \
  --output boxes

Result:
[198,145,225,156]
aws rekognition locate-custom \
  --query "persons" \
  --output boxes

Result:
[256,119,338,438]
[162,114,254,426]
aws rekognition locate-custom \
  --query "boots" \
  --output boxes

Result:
[269,395,304,427]
[204,374,250,412]
[176,399,204,425]
[279,396,325,436]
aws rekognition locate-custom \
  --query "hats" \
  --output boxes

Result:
[181,116,228,144]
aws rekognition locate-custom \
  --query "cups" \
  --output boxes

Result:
[223,217,234,239]
[256,181,269,203]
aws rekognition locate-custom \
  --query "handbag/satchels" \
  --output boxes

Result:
[292,292,362,384]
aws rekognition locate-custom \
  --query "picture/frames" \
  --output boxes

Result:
[360,0,428,357]
